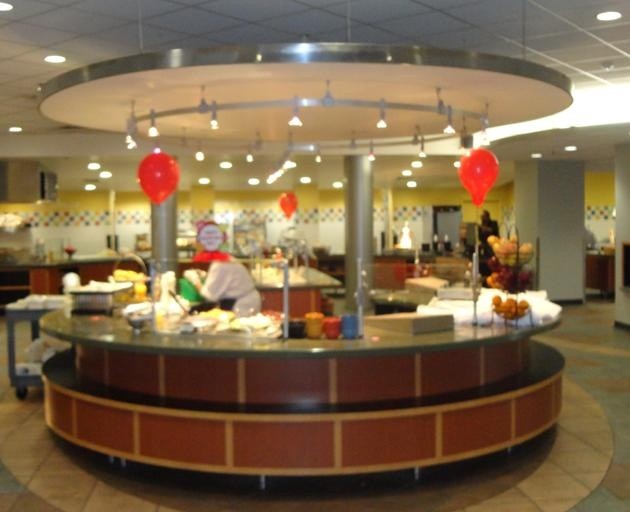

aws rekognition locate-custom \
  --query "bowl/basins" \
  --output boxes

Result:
[282,311,358,340]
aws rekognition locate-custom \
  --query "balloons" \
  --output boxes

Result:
[279,191,298,221]
[137,151,180,206]
[458,148,500,209]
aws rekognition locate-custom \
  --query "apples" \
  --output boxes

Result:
[486,235,533,289]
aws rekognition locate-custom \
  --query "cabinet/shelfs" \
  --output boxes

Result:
[310,255,346,296]
[0,268,61,318]
[372,255,436,298]
[585,253,614,300]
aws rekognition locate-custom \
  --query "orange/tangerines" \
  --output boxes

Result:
[493,295,529,319]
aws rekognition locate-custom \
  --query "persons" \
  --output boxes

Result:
[478,210,499,277]
[183,255,262,317]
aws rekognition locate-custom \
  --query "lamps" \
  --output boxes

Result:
[442,105,457,135]
[288,107,304,127]
[376,105,388,129]
[149,117,160,136]
[210,100,218,127]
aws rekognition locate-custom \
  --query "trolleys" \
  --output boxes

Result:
[5,293,71,399]
